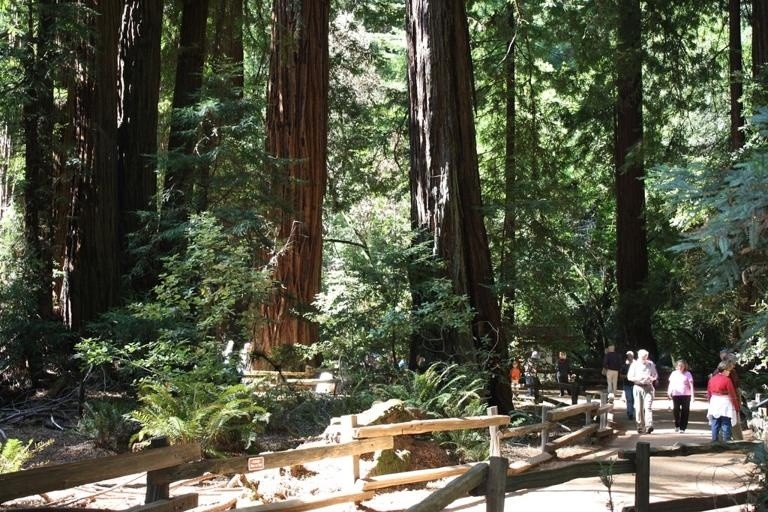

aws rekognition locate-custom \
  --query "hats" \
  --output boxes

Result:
[627,351,634,356]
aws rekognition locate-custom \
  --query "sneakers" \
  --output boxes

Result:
[638,427,644,433]
[675,426,685,434]
[646,426,654,434]
[627,413,634,420]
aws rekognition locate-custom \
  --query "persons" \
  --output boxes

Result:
[706,350,745,443]
[602,345,623,397]
[619,348,659,434]
[555,351,571,397]
[666,358,696,433]
[510,350,541,400]
